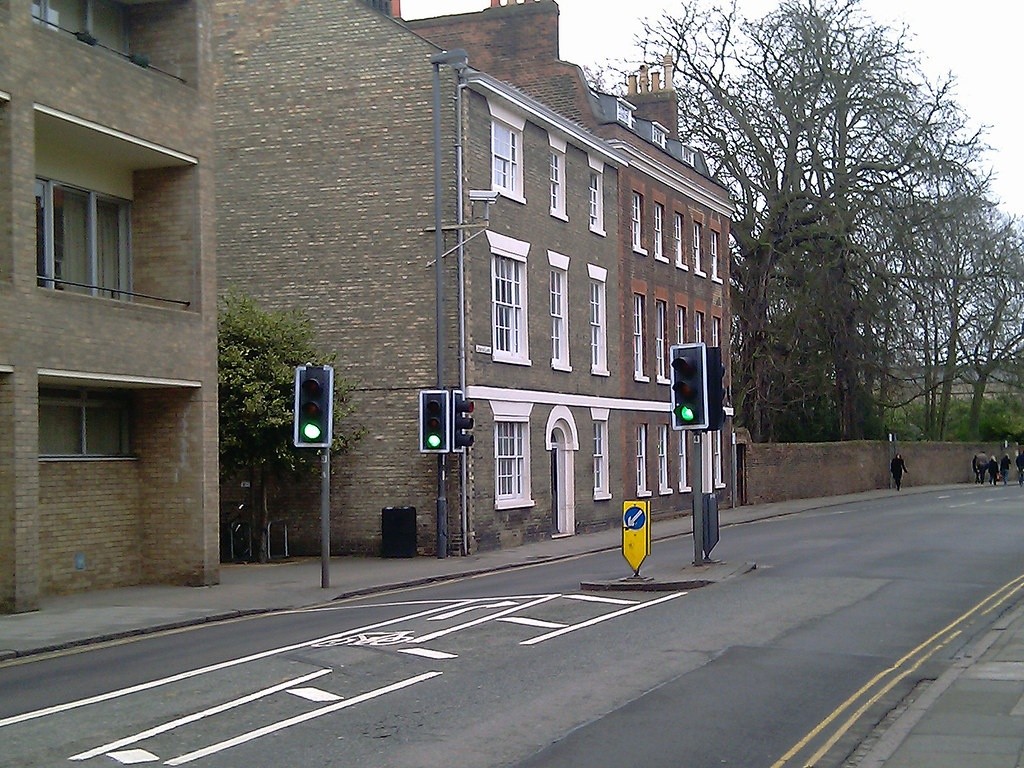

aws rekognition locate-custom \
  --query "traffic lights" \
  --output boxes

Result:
[451,390,475,455]
[418,388,453,453]
[704,347,726,431]
[668,343,710,430]
[295,363,335,448]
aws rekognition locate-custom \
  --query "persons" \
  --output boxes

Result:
[1000,454,1011,487]
[971,450,1000,486]
[1015,450,1024,488]
[890,454,908,491]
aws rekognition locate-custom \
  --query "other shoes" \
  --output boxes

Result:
[994,481,996,485]
[989,481,991,484]
[897,488,899,491]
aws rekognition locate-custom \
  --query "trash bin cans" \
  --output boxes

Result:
[380,505,417,557]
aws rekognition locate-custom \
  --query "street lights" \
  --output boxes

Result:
[430,46,470,557]
